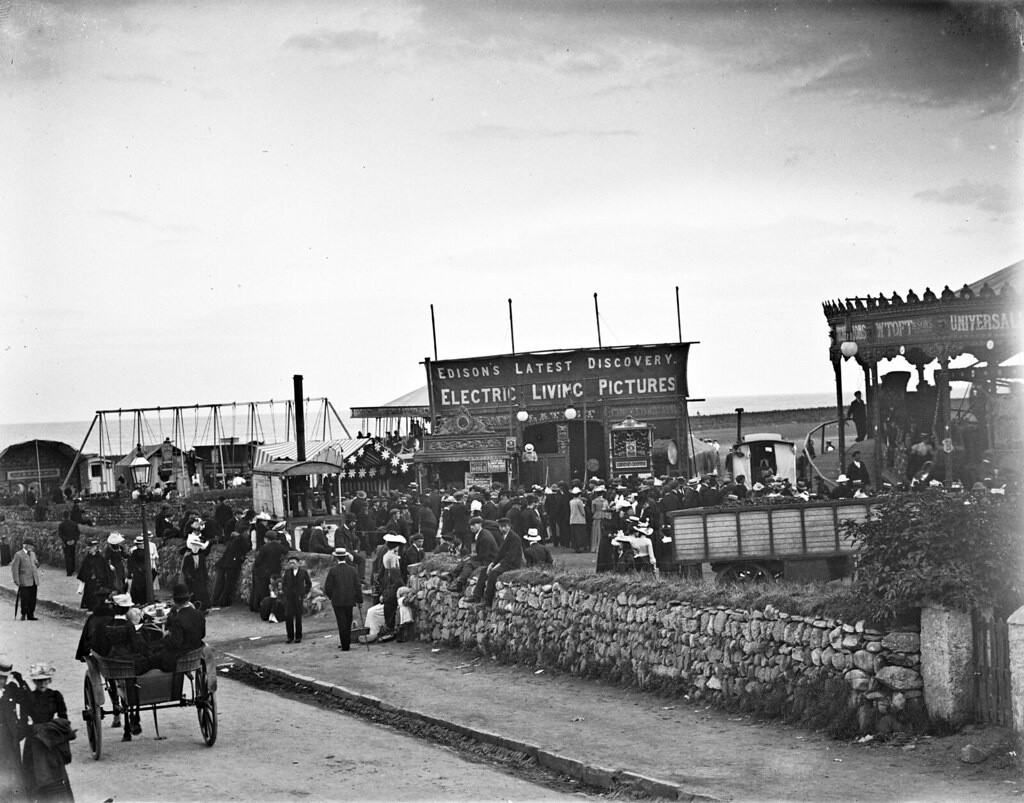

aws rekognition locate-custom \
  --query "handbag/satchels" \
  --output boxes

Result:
[153,574,160,590]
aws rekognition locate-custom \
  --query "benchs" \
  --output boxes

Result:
[87,642,206,683]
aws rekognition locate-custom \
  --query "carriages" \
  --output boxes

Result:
[82,582,218,761]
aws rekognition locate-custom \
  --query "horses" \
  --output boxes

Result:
[83,581,141,742]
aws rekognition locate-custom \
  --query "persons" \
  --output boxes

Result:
[507,440,515,451]
[396,586,418,643]
[282,556,311,643]
[58,511,80,576]
[76,531,206,710]
[357,419,427,454]
[324,548,364,651]
[0,474,177,527]
[523,528,553,567]
[12,538,40,620]
[521,443,538,462]
[700,438,720,455]
[155,450,872,643]
[0,653,79,803]
[884,414,1016,494]
[463,518,523,609]
[436,516,498,592]
[848,391,866,443]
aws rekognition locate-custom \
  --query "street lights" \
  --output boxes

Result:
[130,444,156,605]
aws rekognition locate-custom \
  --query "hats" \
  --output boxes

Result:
[0,661,12,676]
[86,541,99,546]
[272,521,287,531]
[191,518,207,530]
[127,607,144,623]
[30,664,55,679]
[525,443,534,453]
[107,532,125,544]
[241,509,249,517]
[23,537,37,544]
[105,594,135,606]
[257,512,271,520]
[134,536,144,543]
[162,503,172,509]
[141,530,153,537]
[331,473,985,556]
[169,584,193,598]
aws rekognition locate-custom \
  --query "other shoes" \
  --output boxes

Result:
[438,574,453,583]
[447,585,463,593]
[295,639,301,643]
[463,595,481,603]
[476,601,492,608]
[286,637,294,642]
